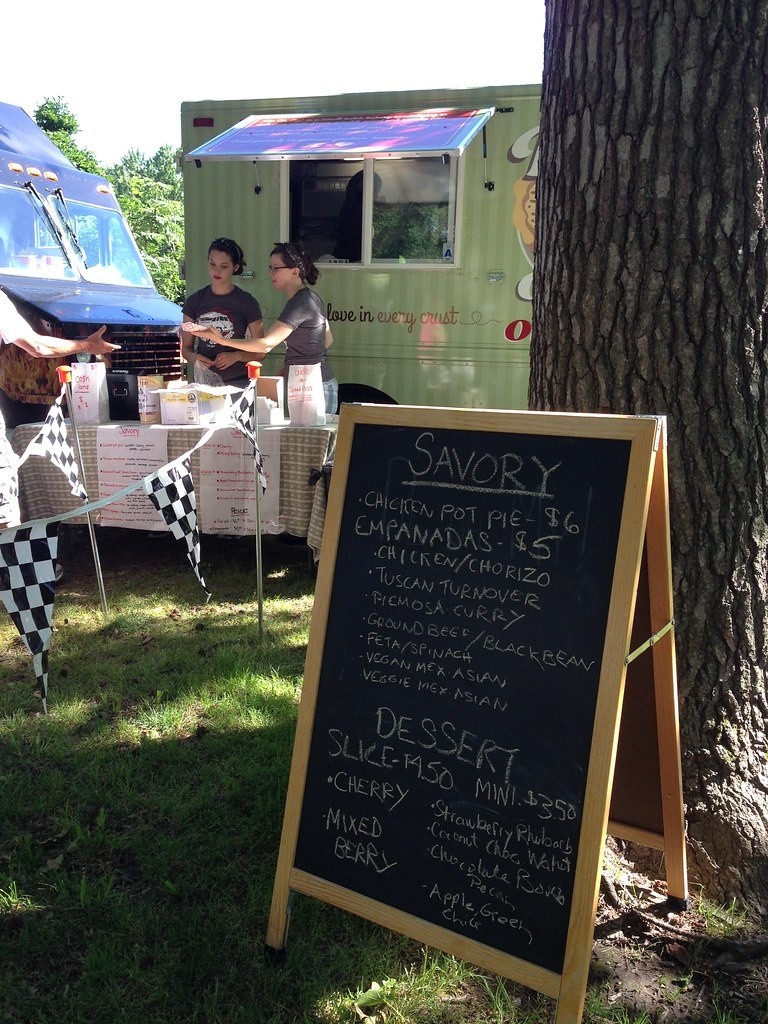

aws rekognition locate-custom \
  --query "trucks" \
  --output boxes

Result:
[0,102,185,430]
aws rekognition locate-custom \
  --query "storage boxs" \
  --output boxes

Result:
[148,384,245,425]
[136,374,164,425]
[256,375,284,426]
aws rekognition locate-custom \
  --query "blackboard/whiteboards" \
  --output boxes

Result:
[268,402,690,1001]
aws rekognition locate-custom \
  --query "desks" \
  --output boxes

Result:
[9,412,341,579]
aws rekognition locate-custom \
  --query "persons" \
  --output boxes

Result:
[190,240,339,415]
[181,237,266,412]
[0,289,121,527]
[335,168,380,260]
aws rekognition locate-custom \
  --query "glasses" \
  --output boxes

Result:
[268,266,295,272]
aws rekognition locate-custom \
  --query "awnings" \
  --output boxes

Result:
[185,106,495,193]
